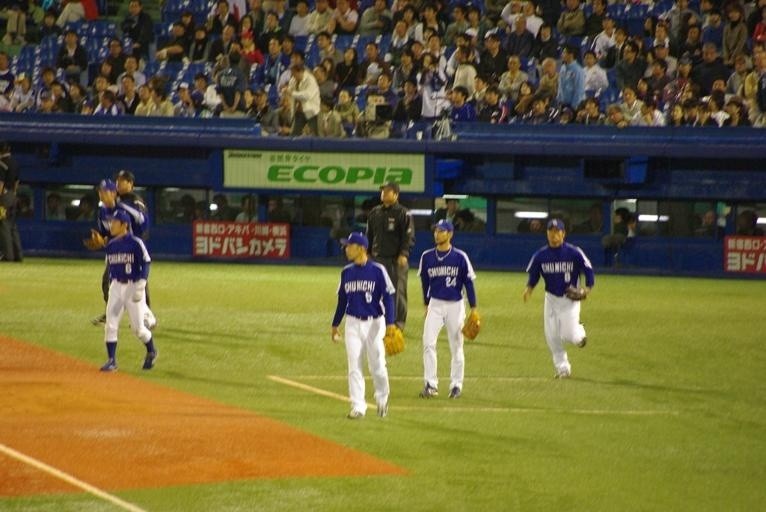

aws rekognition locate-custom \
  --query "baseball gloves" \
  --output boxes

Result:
[462,307,481,339]
[384,326,406,356]
[567,284,589,300]
[83,229,108,251]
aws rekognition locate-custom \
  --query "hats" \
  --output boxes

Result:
[379,181,400,193]
[115,169,136,181]
[340,231,369,249]
[430,219,454,231]
[96,178,116,191]
[105,209,130,221]
[547,219,565,231]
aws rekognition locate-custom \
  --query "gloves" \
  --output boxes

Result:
[136,278,147,290]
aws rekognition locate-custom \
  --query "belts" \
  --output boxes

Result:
[356,316,375,320]
[116,278,139,283]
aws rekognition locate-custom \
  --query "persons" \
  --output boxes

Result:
[331,231,397,419]
[416,220,477,399]
[92,171,152,326]
[0,1,766,143]
[0,144,23,262]
[16,188,764,245]
[90,209,158,371]
[522,218,595,379]
[85,180,159,330]
[365,182,417,332]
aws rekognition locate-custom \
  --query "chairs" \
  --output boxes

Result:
[10,1,753,112]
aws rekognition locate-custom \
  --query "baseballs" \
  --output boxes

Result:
[334,335,341,343]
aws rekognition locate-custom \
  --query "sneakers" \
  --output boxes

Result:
[377,402,388,418]
[346,409,359,418]
[100,358,116,372]
[419,382,438,397]
[577,322,587,348]
[448,387,460,399]
[143,351,157,369]
[554,370,567,379]
